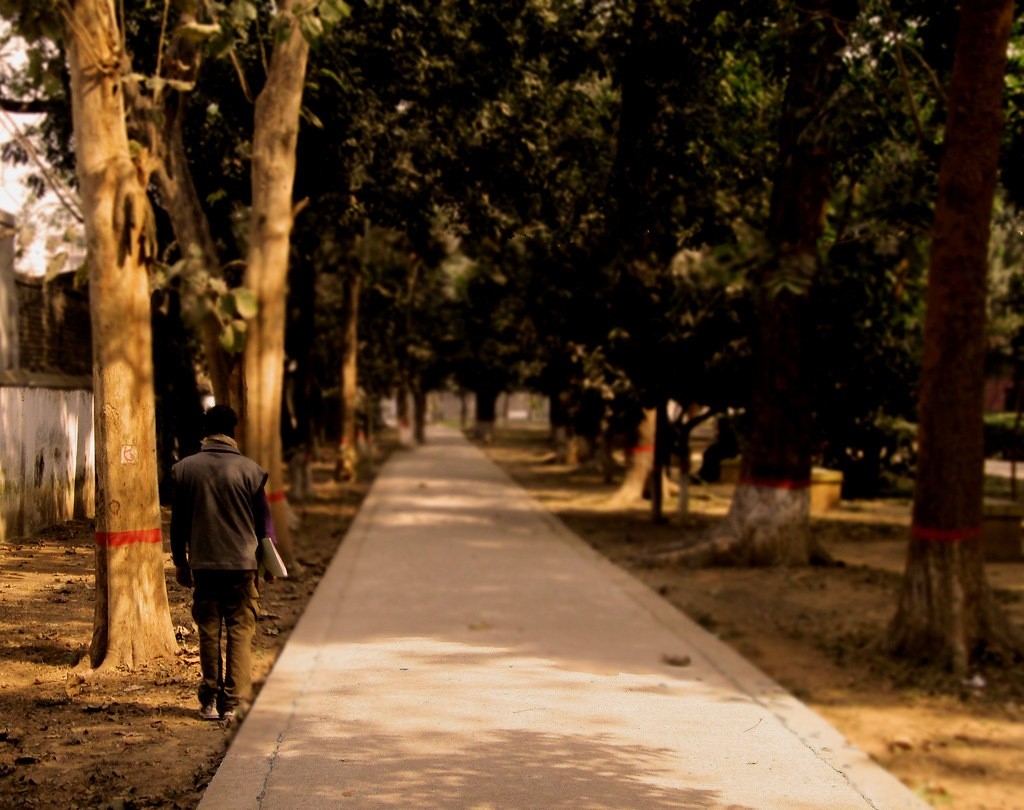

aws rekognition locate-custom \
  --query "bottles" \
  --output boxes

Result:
[968,670,987,702]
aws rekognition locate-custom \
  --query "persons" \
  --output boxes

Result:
[170,405,278,719]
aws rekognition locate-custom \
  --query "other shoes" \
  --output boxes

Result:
[222,708,235,718]
[199,698,219,718]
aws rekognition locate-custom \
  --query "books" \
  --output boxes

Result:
[262,538,288,577]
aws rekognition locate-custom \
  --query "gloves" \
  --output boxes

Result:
[264,571,278,583]
[175,558,194,587]
